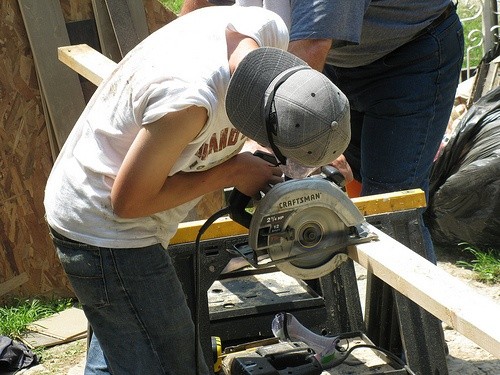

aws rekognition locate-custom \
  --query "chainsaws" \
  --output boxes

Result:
[227,150,378,280]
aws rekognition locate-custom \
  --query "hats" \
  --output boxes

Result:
[225,46,353,164]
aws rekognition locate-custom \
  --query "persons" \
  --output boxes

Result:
[41,5,356,375]
[177,0,463,375]
